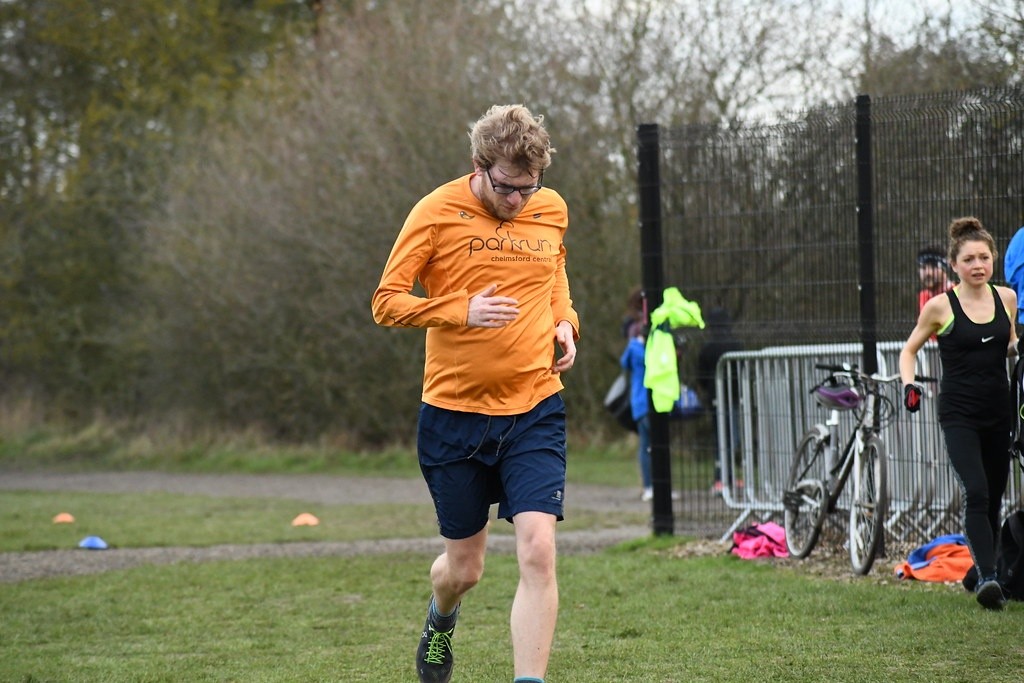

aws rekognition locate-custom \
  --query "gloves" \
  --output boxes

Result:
[904,384,921,412]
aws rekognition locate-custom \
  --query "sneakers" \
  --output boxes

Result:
[975,580,1004,609]
[416,592,461,683]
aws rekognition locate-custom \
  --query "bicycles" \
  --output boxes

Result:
[778,363,940,576]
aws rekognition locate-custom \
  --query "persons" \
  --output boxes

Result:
[1004,225,1024,475]
[917,240,957,340]
[372,103,581,683]
[622,288,685,504]
[898,215,1024,610]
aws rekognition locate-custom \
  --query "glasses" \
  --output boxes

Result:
[484,166,544,195]
